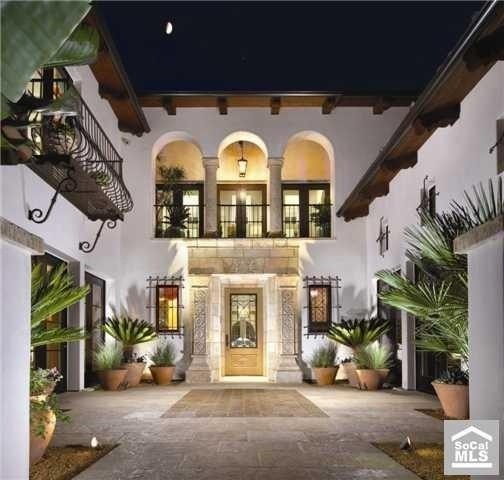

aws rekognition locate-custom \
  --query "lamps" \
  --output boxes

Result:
[237,141,247,177]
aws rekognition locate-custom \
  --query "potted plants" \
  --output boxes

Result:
[89,345,127,391]
[29,261,92,466]
[147,341,176,385]
[161,203,191,237]
[374,205,468,419]
[309,204,330,237]
[308,341,339,385]
[100,314,159,387]
[351,344,397,390]
[327,318,393,387]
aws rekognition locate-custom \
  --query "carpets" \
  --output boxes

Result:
[29,444,119,480]
[369,441,468,479]
